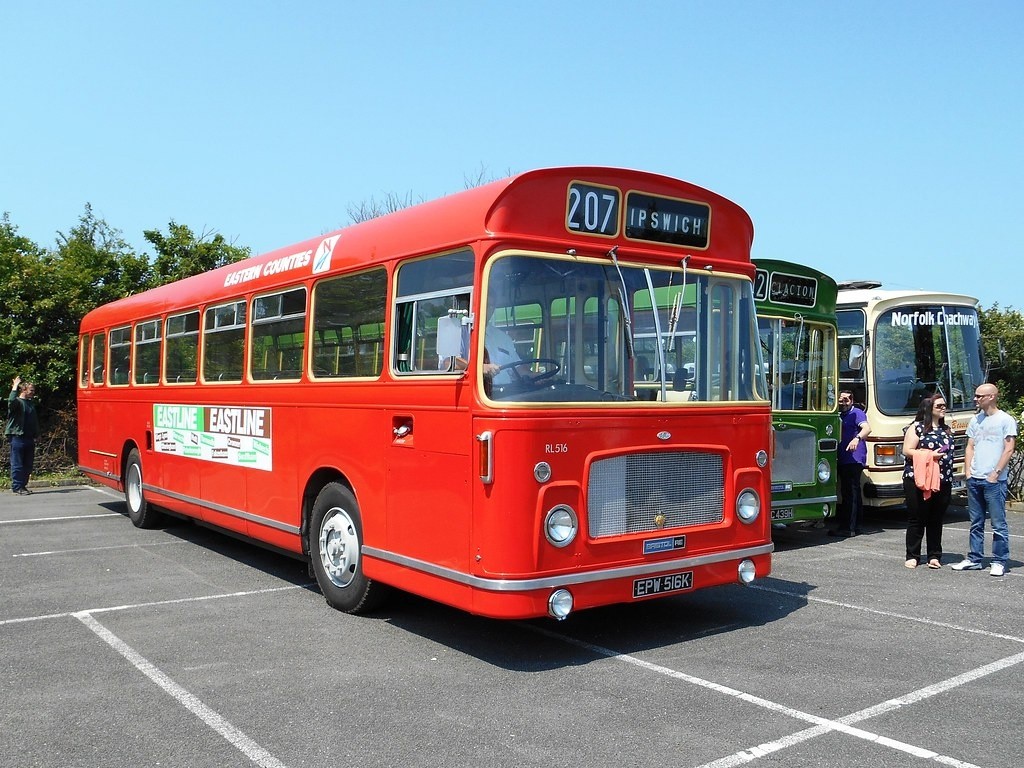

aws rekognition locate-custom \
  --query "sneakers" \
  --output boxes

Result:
[951,559,982,570]
[990,562,1005,576]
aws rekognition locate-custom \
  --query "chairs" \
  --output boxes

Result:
[112,368,296,384]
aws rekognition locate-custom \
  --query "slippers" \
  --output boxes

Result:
[905,559,917,569]
[926,559,940,569]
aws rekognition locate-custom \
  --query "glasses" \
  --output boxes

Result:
[974,393,994,399]
[933,404,947,409]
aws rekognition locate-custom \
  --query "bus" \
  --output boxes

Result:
[825,280,986,526]
[77,166,775,620]
[260,258,840,527]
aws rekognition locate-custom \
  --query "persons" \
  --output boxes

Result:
[438,288,543,390]
[777,373,803,410]
[828,390,872,537]
[4,376,42,496]
[951,384,1018,575]
[902,393,955,569]
[800,518,825,528]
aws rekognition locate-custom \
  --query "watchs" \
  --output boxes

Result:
[994,468,1002,475]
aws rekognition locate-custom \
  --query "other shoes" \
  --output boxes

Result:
[13,486,33,496]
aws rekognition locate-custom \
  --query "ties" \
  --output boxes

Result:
[484,347,493,397]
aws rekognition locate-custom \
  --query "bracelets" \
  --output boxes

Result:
[855,435,861,441]
